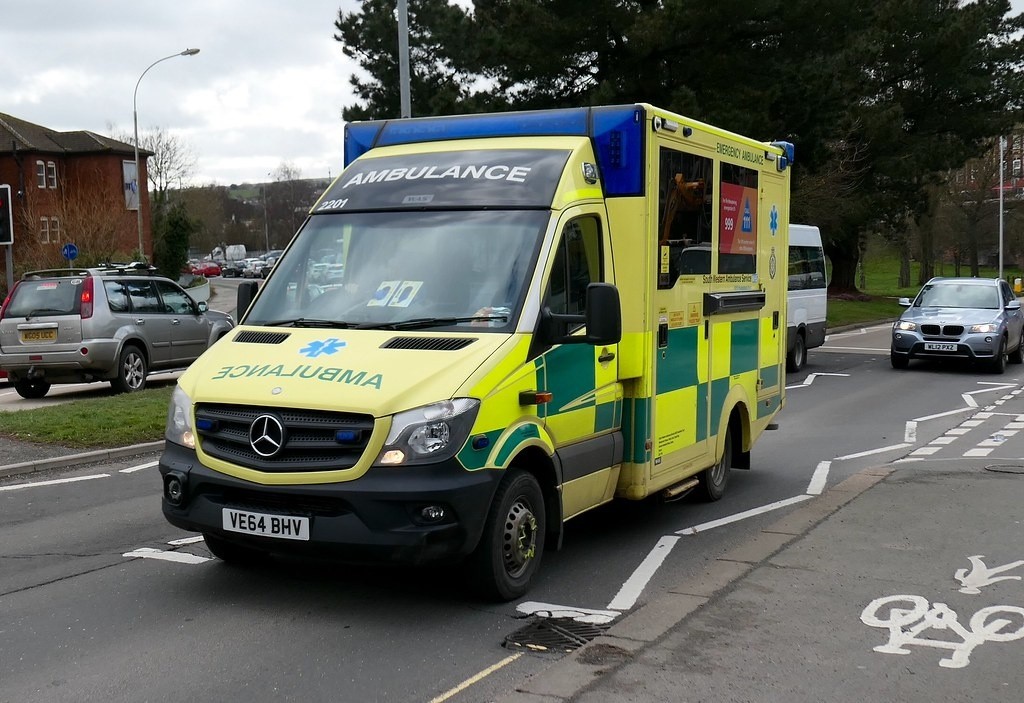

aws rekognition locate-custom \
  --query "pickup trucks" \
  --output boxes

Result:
[255,256,280,278]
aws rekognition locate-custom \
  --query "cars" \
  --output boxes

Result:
[891,277,1024,374]
[258,249,284,264]
[242,261,267,278]
[676,242,756,275]
[242,258,259,263]
[191,261,221,277]
[186,259,201,264]
[312,248,337,264]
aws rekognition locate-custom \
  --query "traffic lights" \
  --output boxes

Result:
[0,184,14,245]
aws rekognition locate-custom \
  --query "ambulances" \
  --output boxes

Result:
[158,101,799,602]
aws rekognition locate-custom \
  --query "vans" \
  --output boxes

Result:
[785,224,828,373]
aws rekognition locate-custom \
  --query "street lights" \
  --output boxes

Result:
[997,135,1008,279]
[134,48,203,262]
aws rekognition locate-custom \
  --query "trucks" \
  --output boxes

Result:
[204,244,247,260]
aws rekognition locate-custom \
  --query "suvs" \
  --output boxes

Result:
[222,260,246,277]
[320,263,344,283]
[310,264,326,282]
[0,268,237,399]
